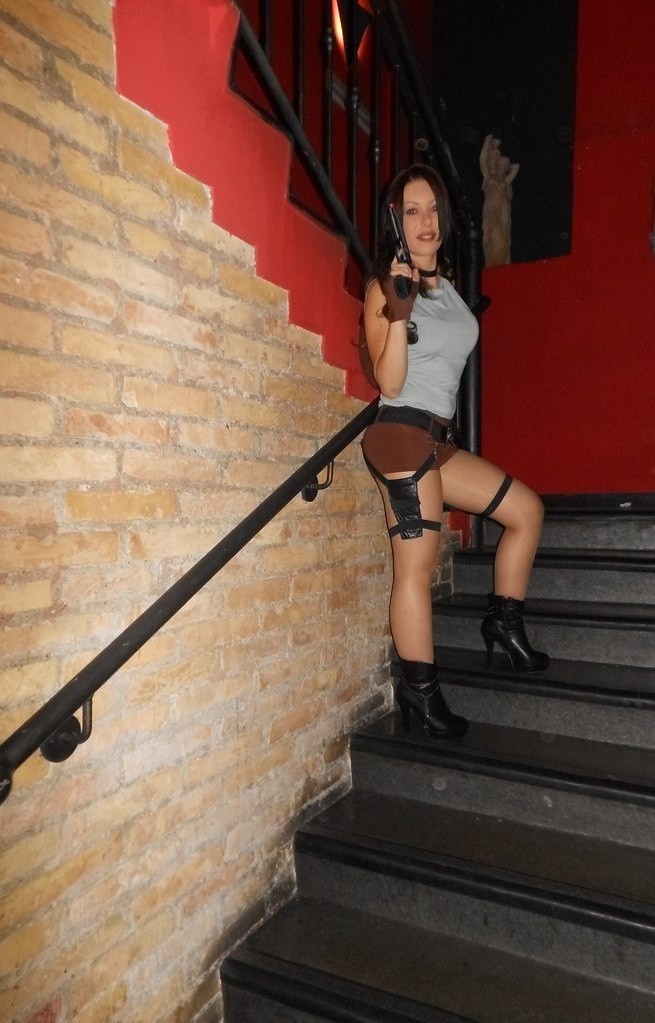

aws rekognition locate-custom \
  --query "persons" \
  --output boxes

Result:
[479,134,519,266]
[360,163,550,743]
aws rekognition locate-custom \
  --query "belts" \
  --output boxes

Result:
[379,406,454,445]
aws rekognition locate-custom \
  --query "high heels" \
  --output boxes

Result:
[481,592,550,673]
[394,658,468,740]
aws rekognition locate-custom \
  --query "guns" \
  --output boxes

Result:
[383,203,416,300]
